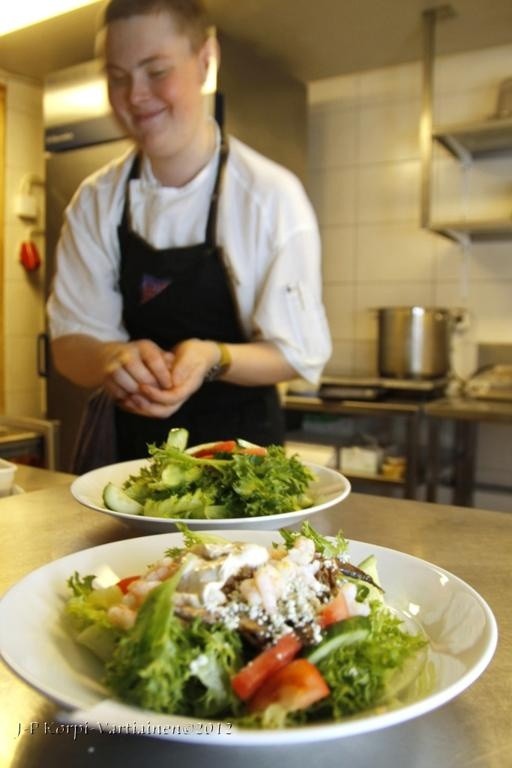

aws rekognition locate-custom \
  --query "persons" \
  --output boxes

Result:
[40,0,335,484]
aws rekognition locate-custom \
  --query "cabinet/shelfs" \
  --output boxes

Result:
[420,7,511,245]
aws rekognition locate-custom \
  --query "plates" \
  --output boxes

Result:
[2,456,498,746]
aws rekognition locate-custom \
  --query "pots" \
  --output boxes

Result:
[375,305,452,380]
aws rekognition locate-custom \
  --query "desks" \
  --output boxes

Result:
[283,376,511,511]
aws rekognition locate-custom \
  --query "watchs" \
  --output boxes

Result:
[204,342,234,384]
